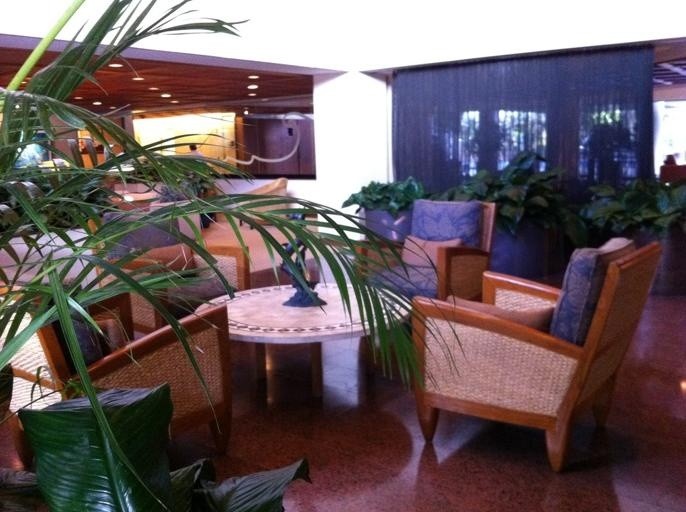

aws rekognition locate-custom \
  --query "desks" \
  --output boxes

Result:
[195,281,415,413]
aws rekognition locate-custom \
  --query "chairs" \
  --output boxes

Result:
[1,209,248,469]
[355,199,661,475]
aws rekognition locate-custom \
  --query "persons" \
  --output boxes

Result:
[663,154,678,165]
[186,144,202,157]
